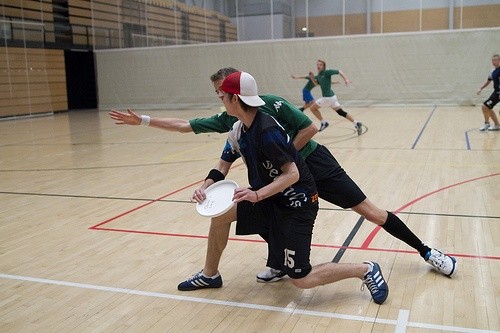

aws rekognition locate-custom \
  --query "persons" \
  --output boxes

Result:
[309,60,363,136]
[476,54,500,131]
[108,67,456,283]
[177,72,389,306]
[291,75,340,112]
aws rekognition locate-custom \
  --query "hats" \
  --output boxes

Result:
[221,71,266,107]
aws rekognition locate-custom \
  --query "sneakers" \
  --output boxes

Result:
[360,261,389,304]
[178,267,223,291]
[256,267,286,283]
[420,245,456,276]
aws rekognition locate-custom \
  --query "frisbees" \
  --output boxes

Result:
[196,179,240,218]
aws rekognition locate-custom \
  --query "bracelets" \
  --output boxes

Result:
[255,190,258,201]
[139,115,150,126]
[205,168,225,183]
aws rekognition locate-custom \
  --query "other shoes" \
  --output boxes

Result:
[479,123,490,131]
[355,121,362,137]
[318,121,329,131]
[488,124,500,130]
